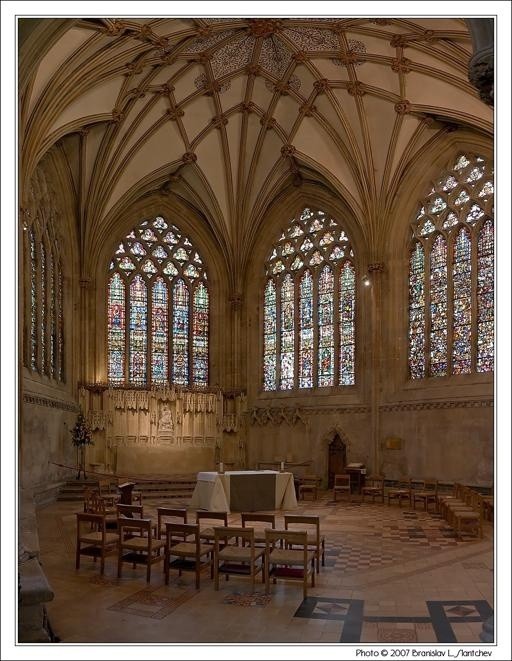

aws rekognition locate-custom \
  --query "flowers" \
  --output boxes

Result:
[70,407,94,448]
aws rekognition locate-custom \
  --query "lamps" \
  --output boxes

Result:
[361,275,370,287]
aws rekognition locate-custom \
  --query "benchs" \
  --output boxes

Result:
[19,556,54,629]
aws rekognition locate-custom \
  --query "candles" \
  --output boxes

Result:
[220,463,223,472]
[281,462,284,470]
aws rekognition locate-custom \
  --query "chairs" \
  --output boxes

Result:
[74,474,324,600]
[334,474,493,538]
